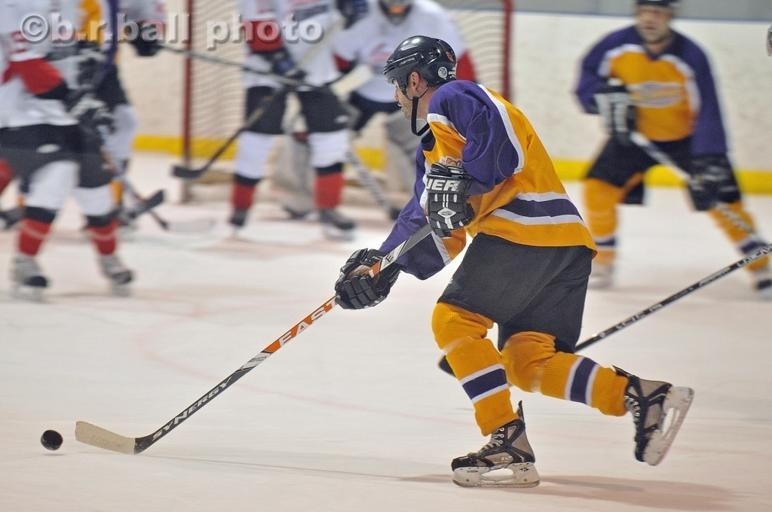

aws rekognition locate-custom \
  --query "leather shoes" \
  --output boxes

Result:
[684,159,726,210]
[259,48,304,81]
[125,22,163,56]
[432,167,476,239]
[63,85,114,137]
[596,91,638,142]
[336,248,400,309]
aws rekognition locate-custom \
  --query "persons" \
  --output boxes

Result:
[0,1,136,288]
[332,33,674,473]
[0,0,171,233]
[569,1,770,287]
[268,0,476,224]
[225,1,373,233]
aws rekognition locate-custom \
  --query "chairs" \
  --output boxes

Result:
[8,258,46,285]
[609,365,671,460]
[317,212,352,229]
[101,255,132,284]
[453,401,535,467]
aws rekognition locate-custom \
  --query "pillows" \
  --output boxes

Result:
[382,35,457,87]
